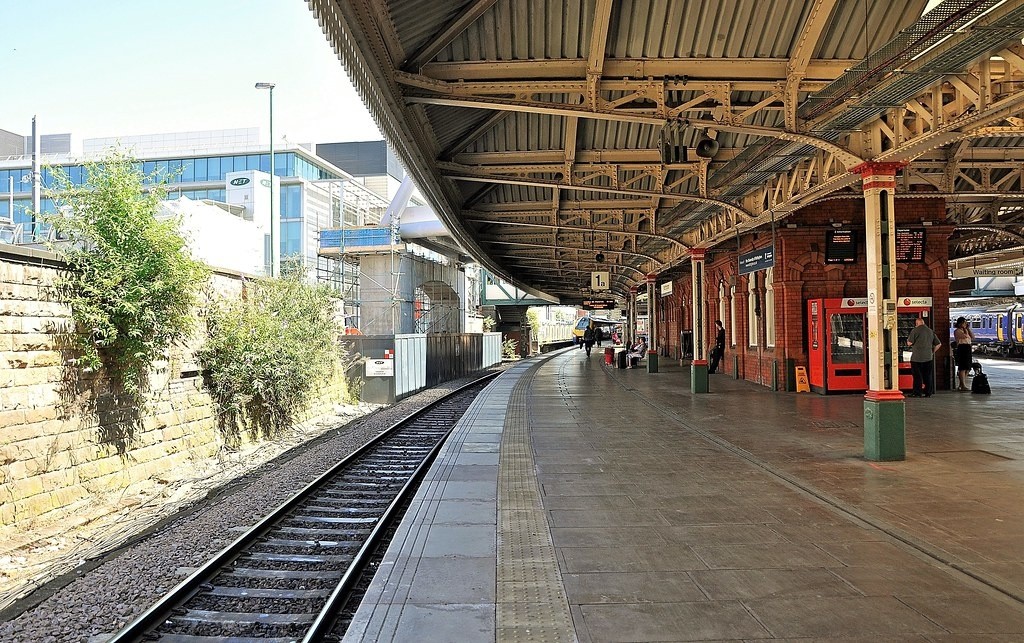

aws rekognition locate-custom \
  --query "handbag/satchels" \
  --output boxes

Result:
[972,373,992,395]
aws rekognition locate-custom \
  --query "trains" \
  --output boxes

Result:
[572,315,619,344]
[949,303,1024,358]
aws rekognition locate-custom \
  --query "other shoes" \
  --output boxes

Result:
[908,393,922,398]
[626,366,632,369]
[925,394,932,398]
[958,385,970,391]
[708,369,715,374]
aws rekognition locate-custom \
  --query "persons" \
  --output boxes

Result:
[907,318,942,398]
[952,316,976,393]
[612,330,619,344]
[626,336,648,369]
[582,326,594,357]
[592,327,603,348]
[707,321,725,374]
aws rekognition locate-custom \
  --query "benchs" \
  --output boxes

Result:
[626,344,647,366]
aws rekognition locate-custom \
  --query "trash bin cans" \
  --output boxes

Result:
[605,348,615,366]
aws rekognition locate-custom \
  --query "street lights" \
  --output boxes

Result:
[255,81,274,281]
